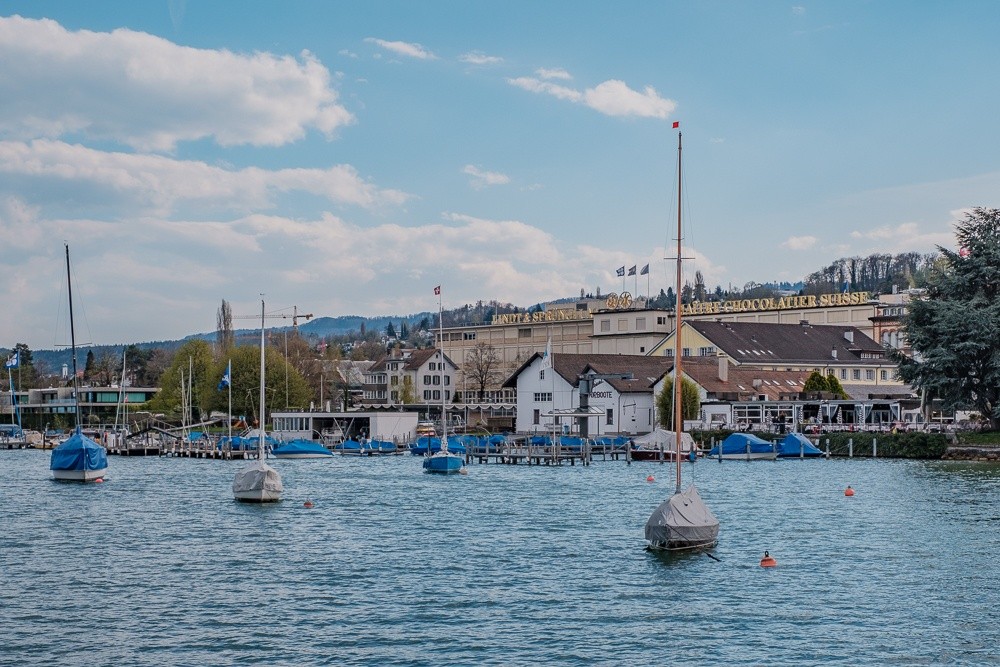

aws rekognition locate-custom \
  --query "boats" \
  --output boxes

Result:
[271,435,332,458]
[774,431,822,458]
[334,439,395,455]
[24,428,57,449]
[623,427,702,461]
[189,431,277,450]
[706,432,778,460]
[410,426,629,456]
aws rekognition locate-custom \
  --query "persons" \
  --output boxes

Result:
[719,410,820,434]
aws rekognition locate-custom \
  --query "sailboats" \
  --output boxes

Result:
[233,292,283,504]
[50,240,107,483]
[0,349,28,450]
[645,130,718,553]
[422,286,465,474]
[104,351,132,446]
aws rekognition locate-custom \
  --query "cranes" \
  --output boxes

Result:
[230,306,314,332]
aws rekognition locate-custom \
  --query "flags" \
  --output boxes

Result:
[844,282,849,291]
[641,265,649,275]
[628,266,637,276]
[218,364,230,390]
[123,396,128,402]
[960,241,971,257]
[434,286,441,295]
[616,267,624,276]
[540,338,552,370]
[4,351,18,370]
